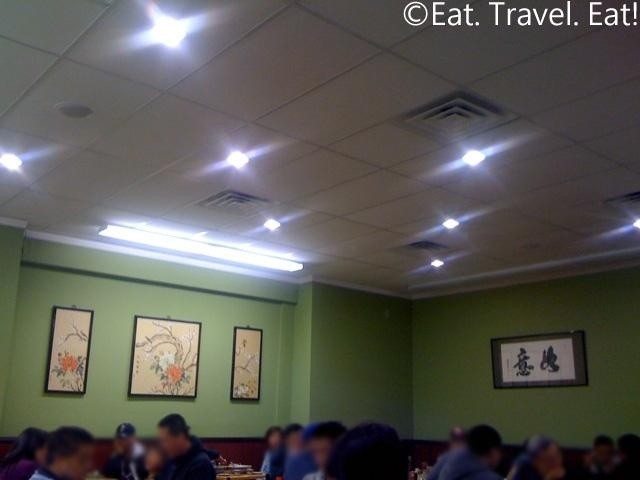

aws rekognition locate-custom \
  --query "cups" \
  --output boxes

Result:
[215,462,256,476]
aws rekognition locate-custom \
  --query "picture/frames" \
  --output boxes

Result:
[128,314,202,396]
[230,326,262,400]
[489,330,588,389]
[45,306,94,394]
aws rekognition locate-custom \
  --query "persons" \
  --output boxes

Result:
[0,412,640,480]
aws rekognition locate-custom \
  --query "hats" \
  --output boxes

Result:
[116,422,135,439]
